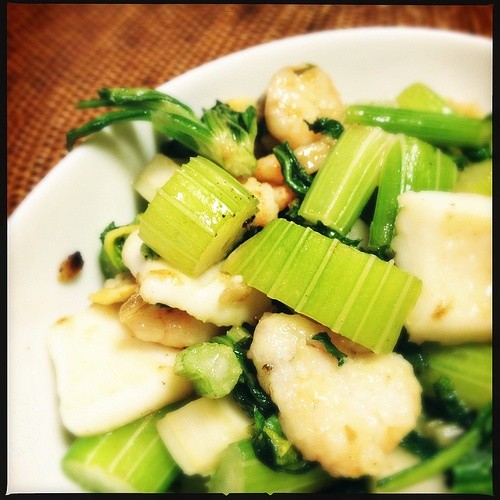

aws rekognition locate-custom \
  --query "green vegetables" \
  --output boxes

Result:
[52,72,495,499]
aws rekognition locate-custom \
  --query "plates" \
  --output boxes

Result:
[6,24,492,495]
